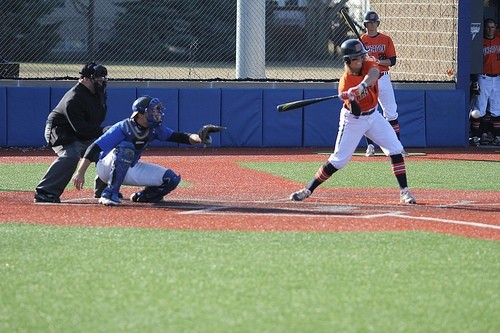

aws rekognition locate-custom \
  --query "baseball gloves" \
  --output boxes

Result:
[198,123,226,142]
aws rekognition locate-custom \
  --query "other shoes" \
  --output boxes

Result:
[130,192,142,202]
[34,193,60,203]
[365,144,375,157]
[290,188,311,202]
[493,136,500,145]
[95,193,123,198]
[401,150,409,156]
[98,187,122,206]
[400,187,416,204]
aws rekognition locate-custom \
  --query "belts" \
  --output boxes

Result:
[481,73,497,77]
[345,104,375,115]
[381,72,388,76]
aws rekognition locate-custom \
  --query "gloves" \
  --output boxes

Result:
[348,84,365,97]
[473,80,481,95]
[338,91,354,101]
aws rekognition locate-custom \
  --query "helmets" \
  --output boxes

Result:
[130,96,151,119]
[341,39,368,63]
[364,11,380,27]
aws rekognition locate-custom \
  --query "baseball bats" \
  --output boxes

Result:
[276,89,368,112]
[341,8,369,57]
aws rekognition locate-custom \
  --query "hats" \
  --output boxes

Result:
[80,63,97,78]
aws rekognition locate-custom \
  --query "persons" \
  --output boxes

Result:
[33,62,123,203]
[474,18,500,146]
[72,95,223,206]
[359,11,410,157]
[290,39,416,204]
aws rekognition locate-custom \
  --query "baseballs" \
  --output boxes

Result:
[471,110,480,118]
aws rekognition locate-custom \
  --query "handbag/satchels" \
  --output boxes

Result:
[49,123,75,147]
